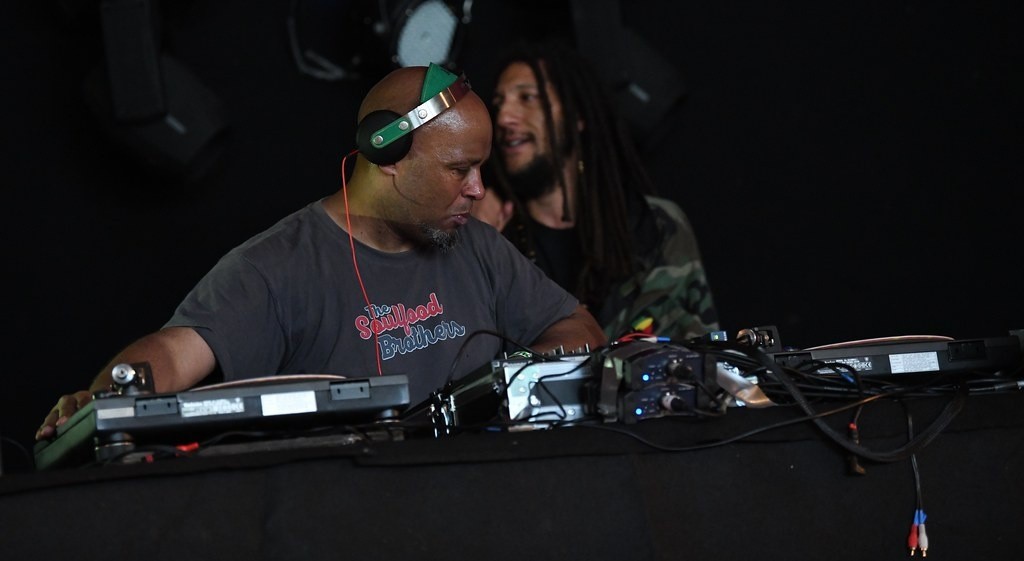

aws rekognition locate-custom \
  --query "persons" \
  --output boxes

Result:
[469,45,722,342]
[33,65,610,445]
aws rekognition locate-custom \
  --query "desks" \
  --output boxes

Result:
[0,388,1024,561]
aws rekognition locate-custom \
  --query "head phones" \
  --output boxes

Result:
[356,70,473,166]
